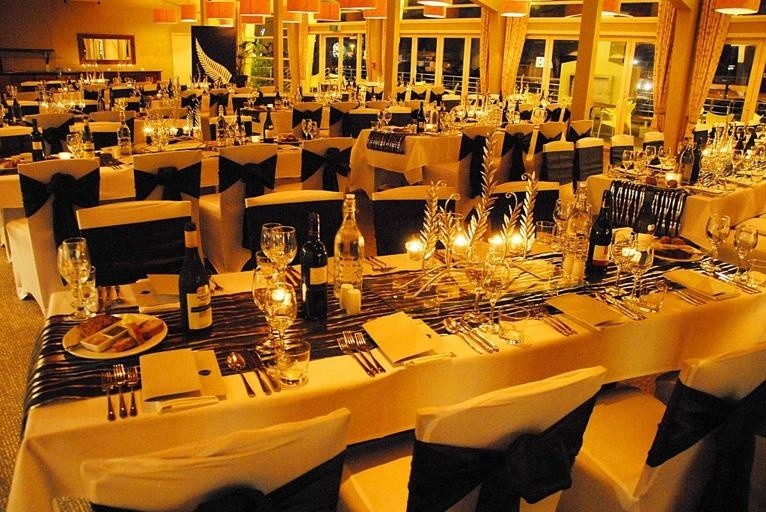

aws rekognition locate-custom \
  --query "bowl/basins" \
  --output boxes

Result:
[23,112,74,129]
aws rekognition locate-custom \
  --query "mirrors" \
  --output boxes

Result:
[76,33,136,65]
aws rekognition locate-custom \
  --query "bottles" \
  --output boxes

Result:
[0,94,22,128]
[30,89,132,162]
[178,222,214,337]
[217,87,281,147]
[606,123,766,196]
[345,78,356,92]
[139,79,174,117]
[371,89,547,135]
[547,263,562,294]
[562,181,612,287]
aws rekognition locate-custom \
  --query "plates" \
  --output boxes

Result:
[274,138,302,144]
[0,158,30,171]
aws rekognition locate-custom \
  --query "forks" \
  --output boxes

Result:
[101,364,138,421]
[338,331,385,377]
[363,256,397,272]
[103,160,123,171]
[658,279,706,306]
[530,307,577,336]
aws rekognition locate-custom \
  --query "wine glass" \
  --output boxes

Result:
[605,228,668,314]
[553,198,572,244]
[705,214,759,283]
[433,213,511,333]
[252,193,366,385]
[56,238,169,360]
[301,118,317,140]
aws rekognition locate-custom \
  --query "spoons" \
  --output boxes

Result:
[208,274,223,291]
[699,260,761,295]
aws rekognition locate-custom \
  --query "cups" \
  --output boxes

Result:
[536,221,556,243]
[497,303,530,344]
[747,258,766,288]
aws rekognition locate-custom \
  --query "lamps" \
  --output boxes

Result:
[152,0,762,27]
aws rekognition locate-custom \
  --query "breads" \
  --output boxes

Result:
[81,311,164,353]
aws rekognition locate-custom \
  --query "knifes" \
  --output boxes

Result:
[227,350,280,395]
[443,317,499,355]
[597,293,645,321]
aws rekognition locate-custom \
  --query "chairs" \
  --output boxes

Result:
[2,72,764,511]
